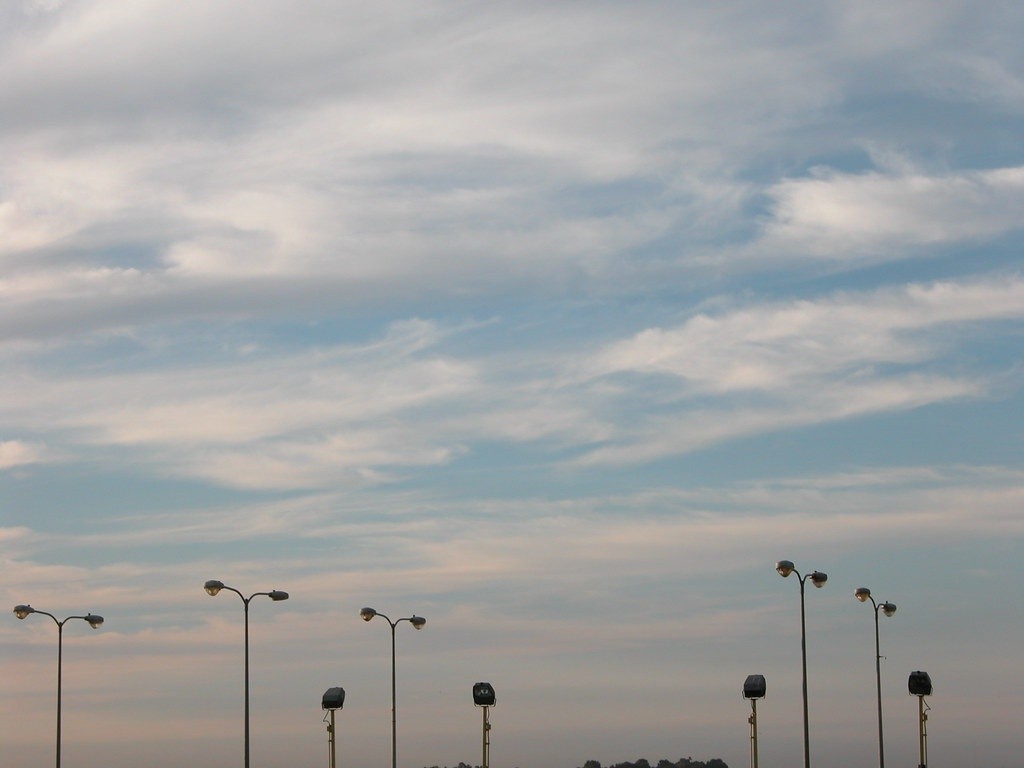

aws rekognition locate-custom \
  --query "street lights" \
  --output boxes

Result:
[14,604,104,768]
[203,580,288,768]
[776,559,829,768]
[322,686,346,767]
[742,673,767,768]
[474,682,497,767]
[908,669,934,768]
[854,587,896,768]
[359,606,427,768]
